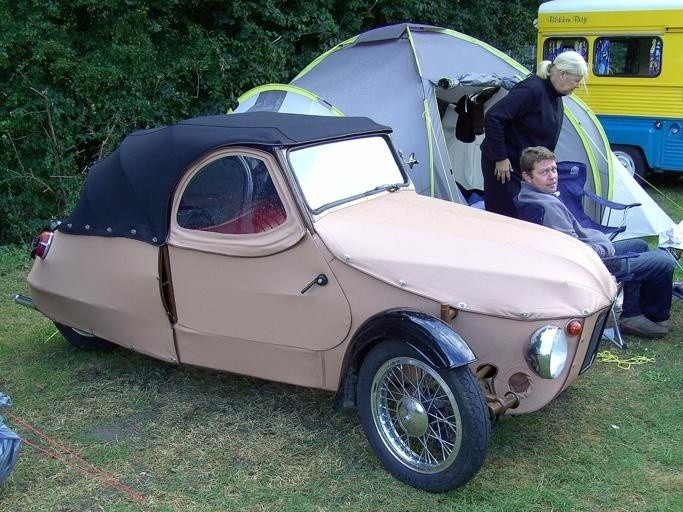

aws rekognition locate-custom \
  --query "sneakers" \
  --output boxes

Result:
[618,313,674,339]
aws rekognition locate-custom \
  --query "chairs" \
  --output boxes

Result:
[194,199,287,235]
[658,246,683,307]
[556,161,642,243]
[513,193,640,348]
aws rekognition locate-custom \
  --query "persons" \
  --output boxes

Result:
[479,50,589,219]
[517,145,676,339]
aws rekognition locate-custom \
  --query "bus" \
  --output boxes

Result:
[529,0,683,188]
